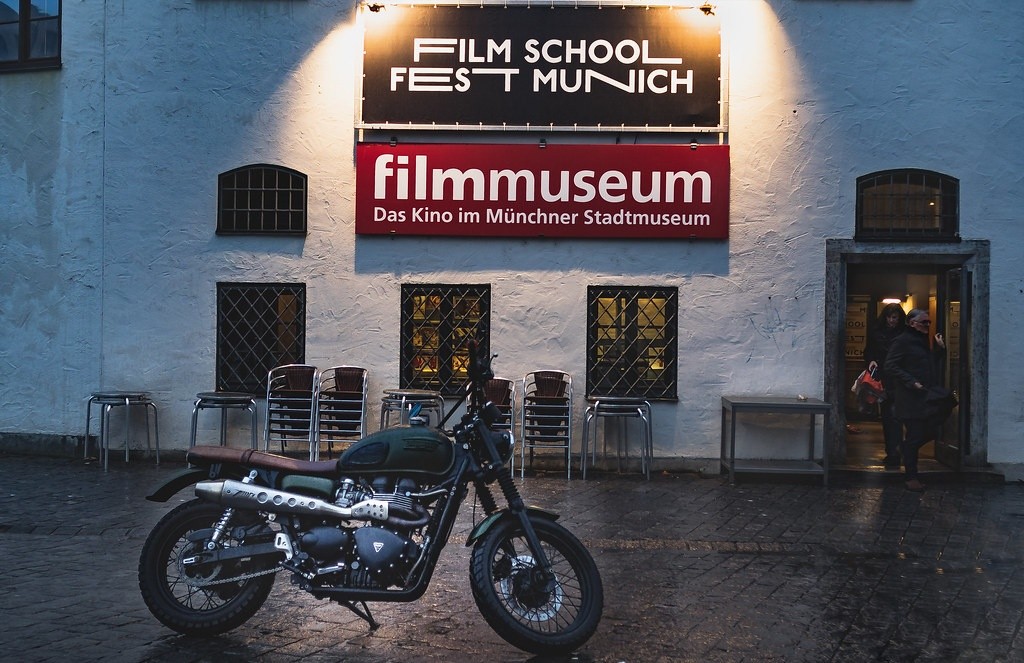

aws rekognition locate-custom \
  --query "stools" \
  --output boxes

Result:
[191,392,259,459]
[579,394,656,479]
[378,386,447,440]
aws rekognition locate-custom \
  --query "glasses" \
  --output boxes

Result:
[913,319,932,327]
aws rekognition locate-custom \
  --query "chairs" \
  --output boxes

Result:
[463,363,571,482]
[263,361,371,462]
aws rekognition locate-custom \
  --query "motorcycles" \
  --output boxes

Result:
[137,340,604,655]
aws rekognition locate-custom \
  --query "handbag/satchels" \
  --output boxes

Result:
[850,364,886,405]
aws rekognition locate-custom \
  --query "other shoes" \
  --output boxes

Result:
[904,478,924,493]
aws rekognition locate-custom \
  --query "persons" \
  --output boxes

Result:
[864,303,907,465]
[885,308,946,491]
